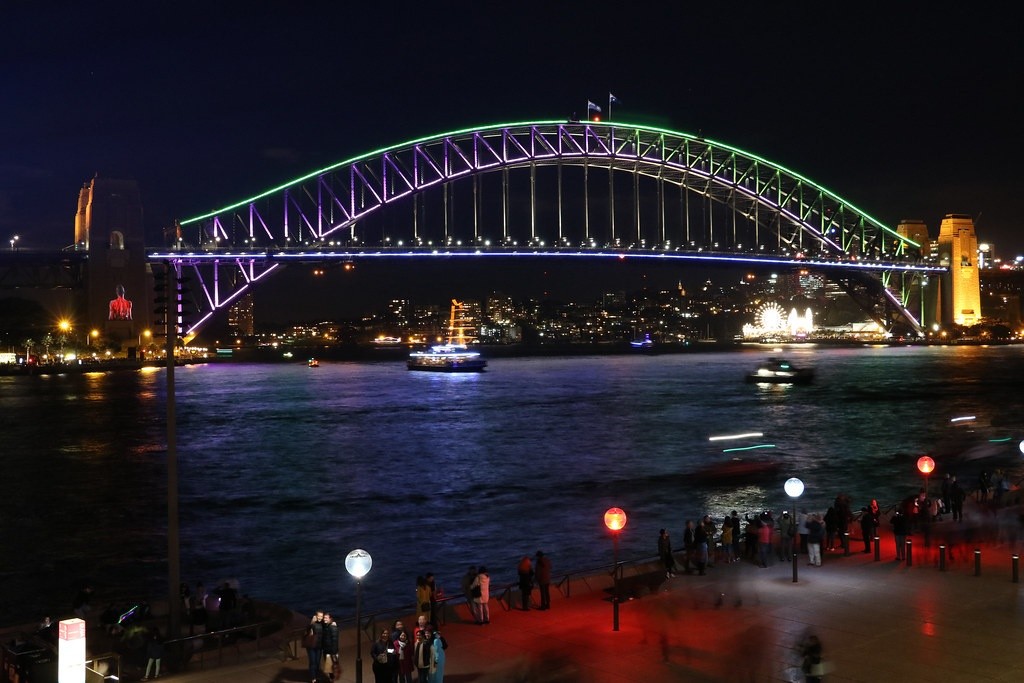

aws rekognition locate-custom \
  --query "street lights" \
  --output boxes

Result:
[86,329,99,355]
[58,320,78,364]
[783,478,805,582]
[343,548,373,683]
[138,329,150,345]
[916,456,935,505]
[604,507,627,630]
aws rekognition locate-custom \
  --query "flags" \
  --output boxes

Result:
[611,94,617,103]
[589,101,601,112]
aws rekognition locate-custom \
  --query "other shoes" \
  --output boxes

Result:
[486,621,490,624]
[480,622,485,625]
[670,574,676,578]
[665,572,670,579]
[538,606,549,610]
[154,674,161,678]
[141,678,148,681]
[736,557,740,561]
[699,572,706,575]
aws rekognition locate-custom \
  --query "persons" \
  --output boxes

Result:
[534,551,551,610]
[860,500,880,553]
[657,529,675,578]
[371,616,446,683]
[518,558,534,611]
[95,603,121,634]
[777,494,852,567]
[140,631,162,681]
[801,636,822,683]
[108,284,133,320]
[195,582,254,618]
[307,610,340,683]
[415,573,435,625]
[978,469,1004,492]
[462,566,489,624]
[683,510,775,577]
[889,509,908,560]
[940,474,966,522]
[905,496,918,536]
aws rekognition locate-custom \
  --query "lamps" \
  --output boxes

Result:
[843,531,850,535]
[1012,554,1019,559]
[939,544,945,548]
[974,548,981,554]
[906,539,912,544]
[874,535,880,539]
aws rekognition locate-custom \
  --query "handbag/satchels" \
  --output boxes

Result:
[377,654,387,664]
[319,652,332,674]
[421,602,430,611]
[332,653,339,662]
[301,625,319,647]
[472,575,482,597]
[787,517,796,536]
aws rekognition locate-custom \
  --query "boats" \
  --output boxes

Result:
[308,358,320,368]
[405,344,488,372]
